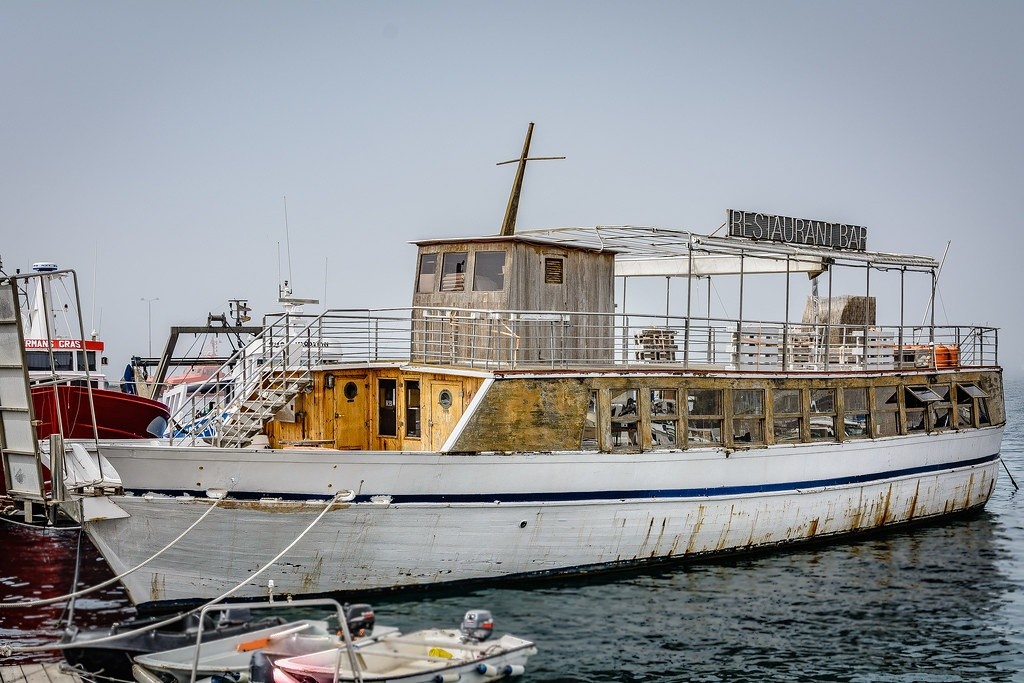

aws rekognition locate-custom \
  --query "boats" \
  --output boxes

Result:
[34,120,1007,613]
[131,604,400,683]
[59,606,280,679]
[273,609,539,683]
[20,262,171,495]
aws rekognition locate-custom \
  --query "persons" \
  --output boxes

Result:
[618,398,637,446]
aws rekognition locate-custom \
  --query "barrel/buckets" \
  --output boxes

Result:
[934,342,961,367]
[893,342,912,350]
[913,342,930,351]
